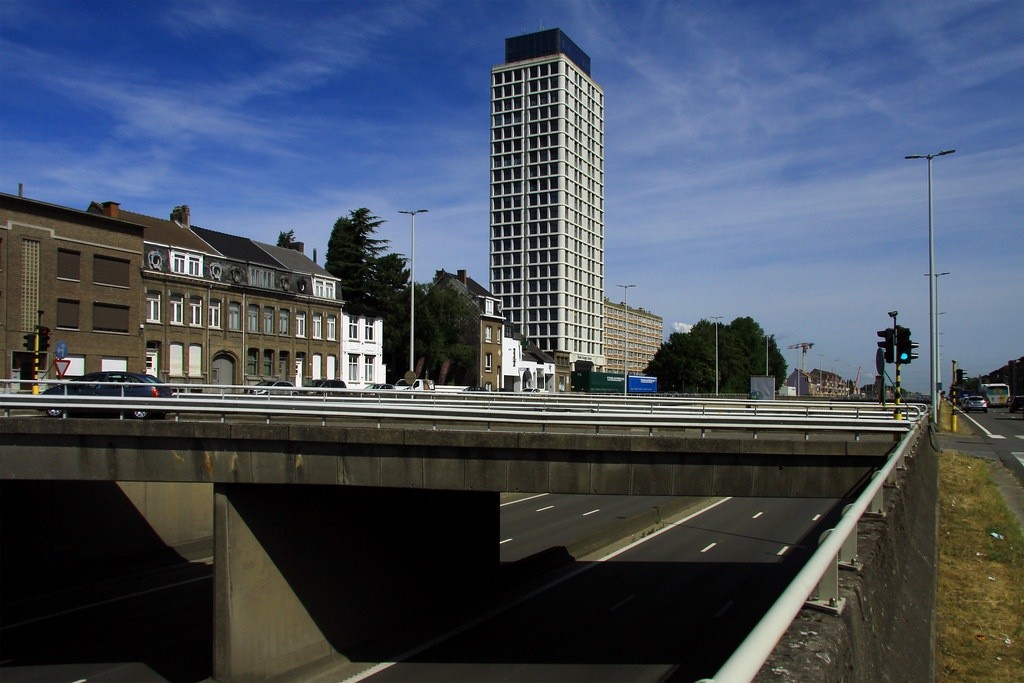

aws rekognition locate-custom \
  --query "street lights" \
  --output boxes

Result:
[709,316,725,397]
[797,344,803,397]
[921,271,952,411]
[764,333,773,376]
[615,284,638,399]
[905,149,958,428]
[398,210,429,376]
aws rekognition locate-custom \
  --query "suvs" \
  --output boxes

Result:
[300,379,348,395]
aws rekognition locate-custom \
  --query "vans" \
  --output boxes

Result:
[393,378,435,392]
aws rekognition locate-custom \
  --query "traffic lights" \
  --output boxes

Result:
[898,324,920,366]
[876,327,895,366]
[39,327,50,355]
[22,333,37,351]
[957,368,968,385]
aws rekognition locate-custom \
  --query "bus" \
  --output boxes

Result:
[981,383,1011,408]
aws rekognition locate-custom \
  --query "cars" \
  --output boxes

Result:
[965,396,988,414]
[518,387,542,396]
[35,370,173,420]
[245,381,299,396]
[457,386,488,395]
[357,384,396,397]
[1009,396,1024,414]
[961,397,969,410]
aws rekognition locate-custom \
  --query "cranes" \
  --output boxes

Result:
[788,343,816,373]
